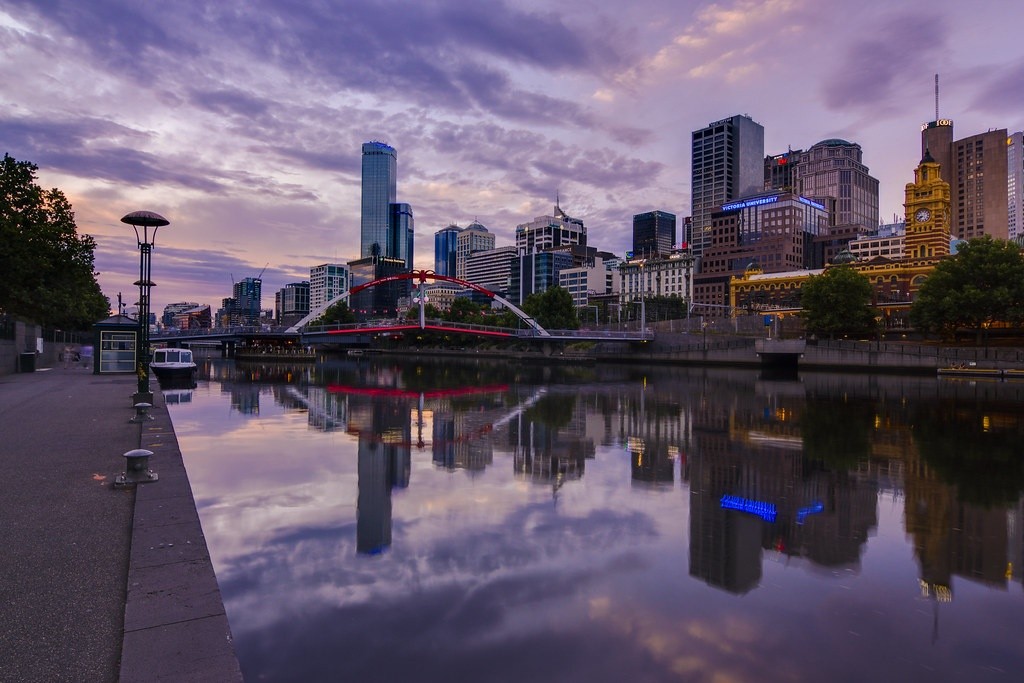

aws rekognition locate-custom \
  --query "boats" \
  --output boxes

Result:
[149,348,198,378]
[936,368,1001,376]
[1002,370,1024,376]
[157,377,197,407]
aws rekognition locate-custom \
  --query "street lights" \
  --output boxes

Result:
[120,210,171,407]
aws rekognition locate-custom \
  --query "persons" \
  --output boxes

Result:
[248,342,312,355]
[63,342,94,370]
[948,361,967,369]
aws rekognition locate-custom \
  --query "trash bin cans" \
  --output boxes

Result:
[20,350,39,372]
